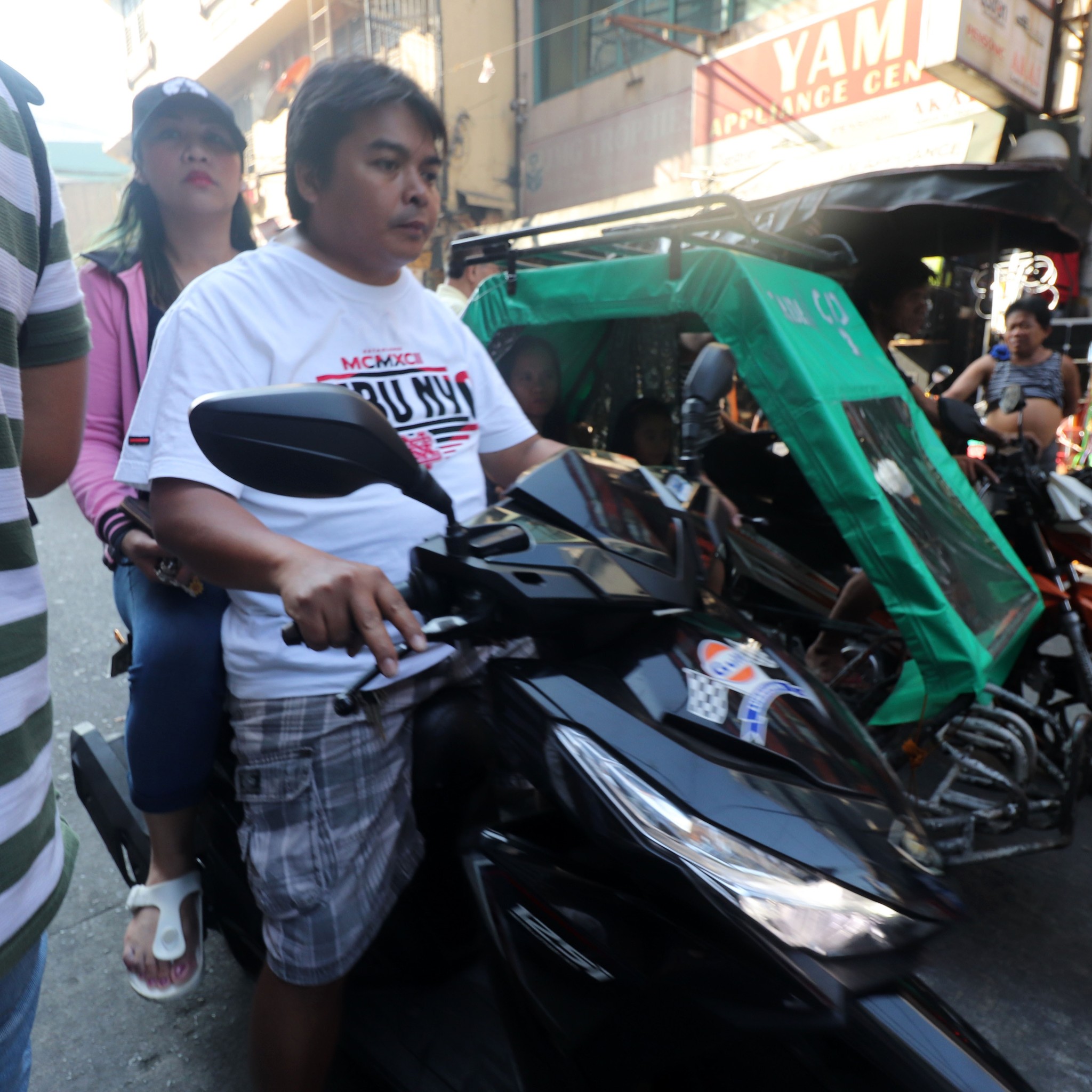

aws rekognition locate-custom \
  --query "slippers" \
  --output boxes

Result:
[124,872,204,1003]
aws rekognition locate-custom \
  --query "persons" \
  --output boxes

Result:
[67,78,262,1004]
[0,62,92,1092]
[435,232,1092,685]
[112,55,570,1092]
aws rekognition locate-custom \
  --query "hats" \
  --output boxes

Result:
[131,76,248,162]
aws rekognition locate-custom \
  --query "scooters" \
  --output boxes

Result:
[68,339,1034,1092]
[451,246,1092,878]
